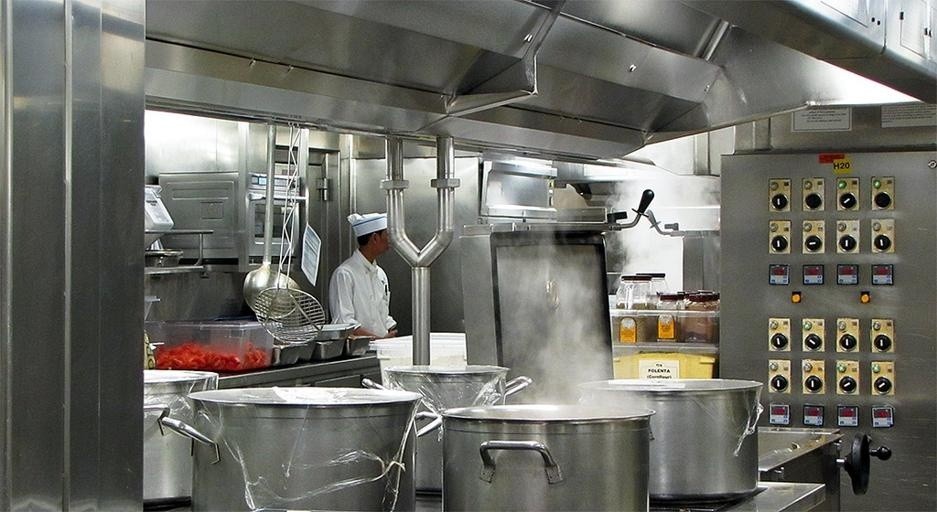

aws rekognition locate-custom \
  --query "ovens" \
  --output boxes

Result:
[158,171,302,260]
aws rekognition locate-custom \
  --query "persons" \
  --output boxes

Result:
[327,210,399,345]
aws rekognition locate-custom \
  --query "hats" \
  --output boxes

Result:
[346,212,387,238]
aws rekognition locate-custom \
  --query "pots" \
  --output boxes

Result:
[146,368,222,504]
[574,376,765,504]
[161,387,424,511]
[360,362,534,494]
[438,403,657,512]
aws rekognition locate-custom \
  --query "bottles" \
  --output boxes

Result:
[611,271,720,344]
